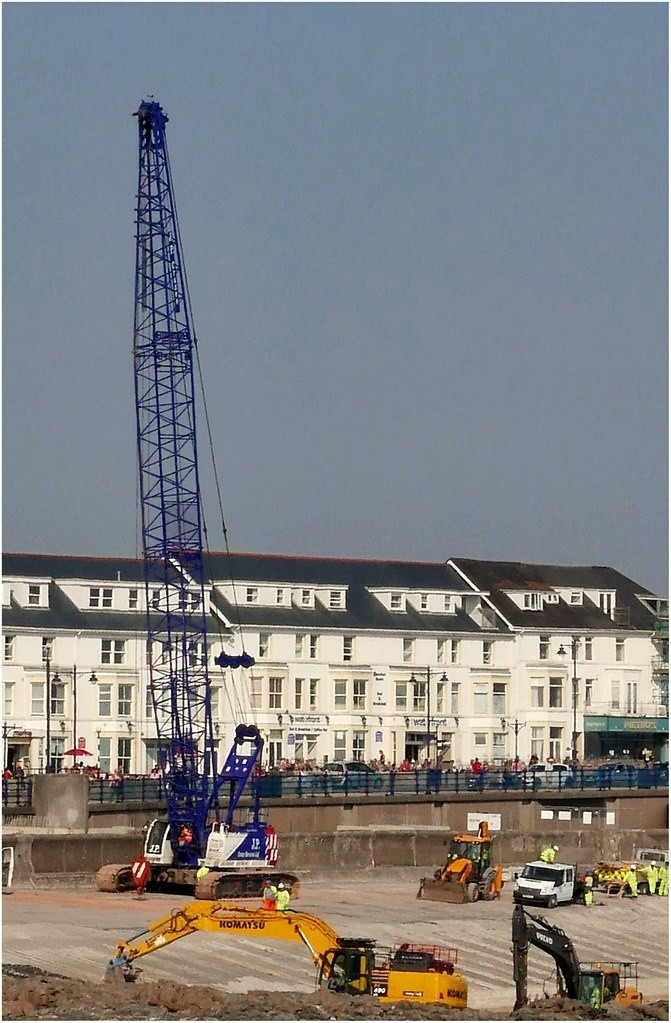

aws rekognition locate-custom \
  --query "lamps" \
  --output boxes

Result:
[502,718,506,728]
[214,722,219,732]
[361,715,366,724]
[128,723,132,732]
[455,717,459,726]
[405,717,410,727]
[61,721,65,730]
[277,713,282,723]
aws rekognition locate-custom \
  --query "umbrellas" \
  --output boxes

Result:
[64,748,93,762]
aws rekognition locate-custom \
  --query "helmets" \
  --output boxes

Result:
[277,883,285,888]
[631,865,636,870]
[586,871,593,875]
[265,879,271,884]
[554,845,559,851]
[650,860,656,865]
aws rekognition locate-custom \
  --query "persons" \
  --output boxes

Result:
[637,859,659,895]
[108,765,126,804]
[361,750,432,771]
[3,766,25,781]
[646,755,656,771]
[463,845,480,860]
[265,757,321,776]
[149,763,167,780]
[658,861,669,896]
[623,865,640,898]
[449,757,491,774]
[573,869,593,905]
[503,752,539,773]
[59,762,97,776]
[544,758,555,789]
[539,844,560,864]
[564,755,582,768]
[262,879,290,910]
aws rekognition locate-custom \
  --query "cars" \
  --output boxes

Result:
[519,763,573,790]
[321,761,385,791]
[586,764,638,787]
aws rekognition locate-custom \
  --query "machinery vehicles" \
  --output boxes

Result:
[512,903,643,1013]
[414,820,504,904]
[103,899,469,1010]
[94,94,300,906]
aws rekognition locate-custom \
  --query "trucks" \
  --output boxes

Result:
[513,860,599,910]
[599,847,669,896]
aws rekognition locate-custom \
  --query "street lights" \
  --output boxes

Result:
[407,664,449,795]
[556,639,578,789]
[51,664,99,767]
